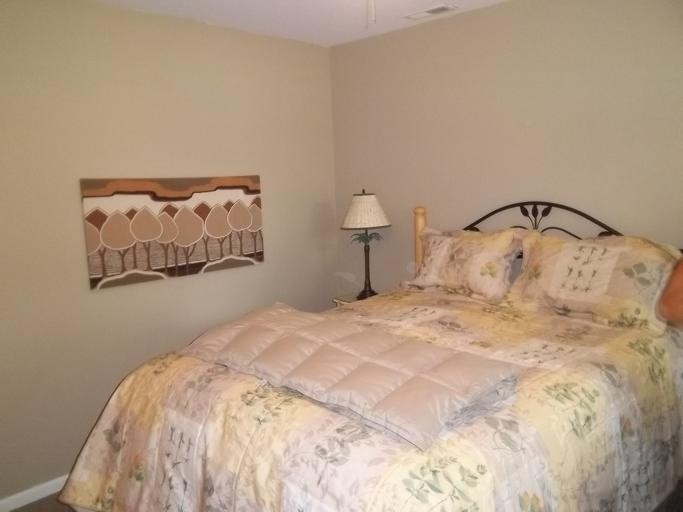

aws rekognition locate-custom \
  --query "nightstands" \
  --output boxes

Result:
[332,291,382,304]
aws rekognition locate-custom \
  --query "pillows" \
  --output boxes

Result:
[410,232,679,334]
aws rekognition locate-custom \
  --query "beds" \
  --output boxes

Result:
[107,201,682,512]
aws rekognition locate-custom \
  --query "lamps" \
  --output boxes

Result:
[340,189,392,300]
